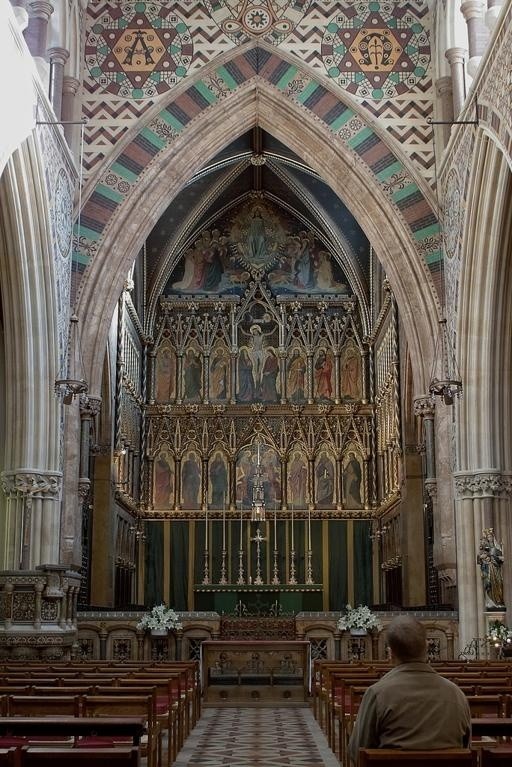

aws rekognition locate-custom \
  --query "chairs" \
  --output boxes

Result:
[0,658,201,766]
[311,658,512,767]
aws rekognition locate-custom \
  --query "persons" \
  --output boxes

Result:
[238,325,277,377]
[344,613,473,766]
[475,526,507,611]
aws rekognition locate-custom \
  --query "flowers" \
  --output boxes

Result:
[136,605,184,631]
[337,603,385,632]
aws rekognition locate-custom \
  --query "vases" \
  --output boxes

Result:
[350,626,367,635]
[150,627,168,635]
